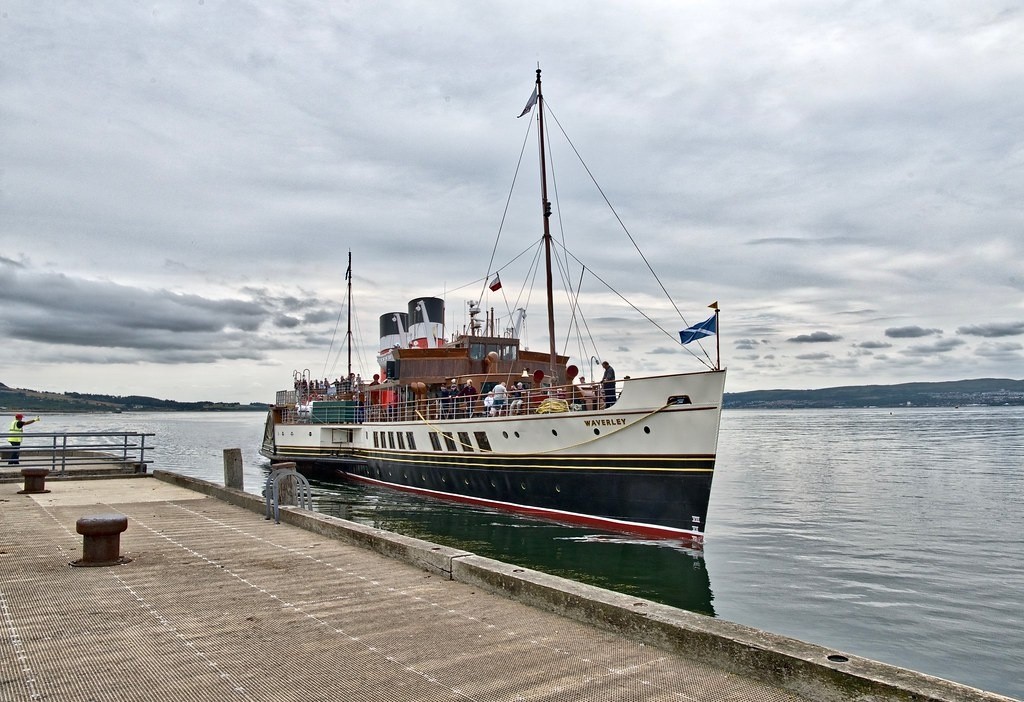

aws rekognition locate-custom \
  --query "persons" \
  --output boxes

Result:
[438,361,617,417]
[295,374,380,405]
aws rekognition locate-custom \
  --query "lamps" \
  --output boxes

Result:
[521,368,529,378]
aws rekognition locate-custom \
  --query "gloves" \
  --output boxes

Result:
[34,415,40,422]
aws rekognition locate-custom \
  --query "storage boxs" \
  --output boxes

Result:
[312,401,363,422]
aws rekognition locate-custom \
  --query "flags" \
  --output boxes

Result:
[488,275,501,292]
[679,313,717,345]
[517,84,537,118]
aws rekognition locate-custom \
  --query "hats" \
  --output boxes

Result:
[579,376,585,379]
[624,376,631,379]
[16,414,24,419]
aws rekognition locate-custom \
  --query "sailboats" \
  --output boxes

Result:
[258,61,728,545]
